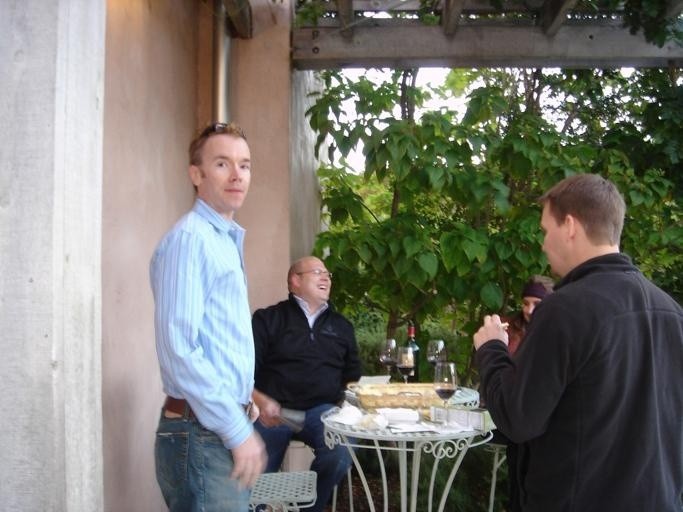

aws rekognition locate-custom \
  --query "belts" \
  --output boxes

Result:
[163,395,253,421]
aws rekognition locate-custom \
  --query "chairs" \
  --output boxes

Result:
[248,471,318,512]
[289,441,338,512]
[484,444,511,512]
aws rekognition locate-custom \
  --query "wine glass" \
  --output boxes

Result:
[378,337,398,384]
[433,361,458,432]
[394,346,416,384]
[425,339,448,383]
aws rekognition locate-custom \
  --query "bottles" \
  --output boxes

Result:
[401,325,421,383]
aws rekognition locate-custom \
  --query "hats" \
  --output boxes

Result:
[519,275,553,300]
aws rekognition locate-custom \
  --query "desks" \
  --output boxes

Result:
[320,406,497,512]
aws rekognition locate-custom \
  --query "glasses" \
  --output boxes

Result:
[296,266,334,279]
[202,122,226,134]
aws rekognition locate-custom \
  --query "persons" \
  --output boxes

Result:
[147,119,281,510]
[472,173,682,511]
[477,273,551,383]
[244,256,370,512]
